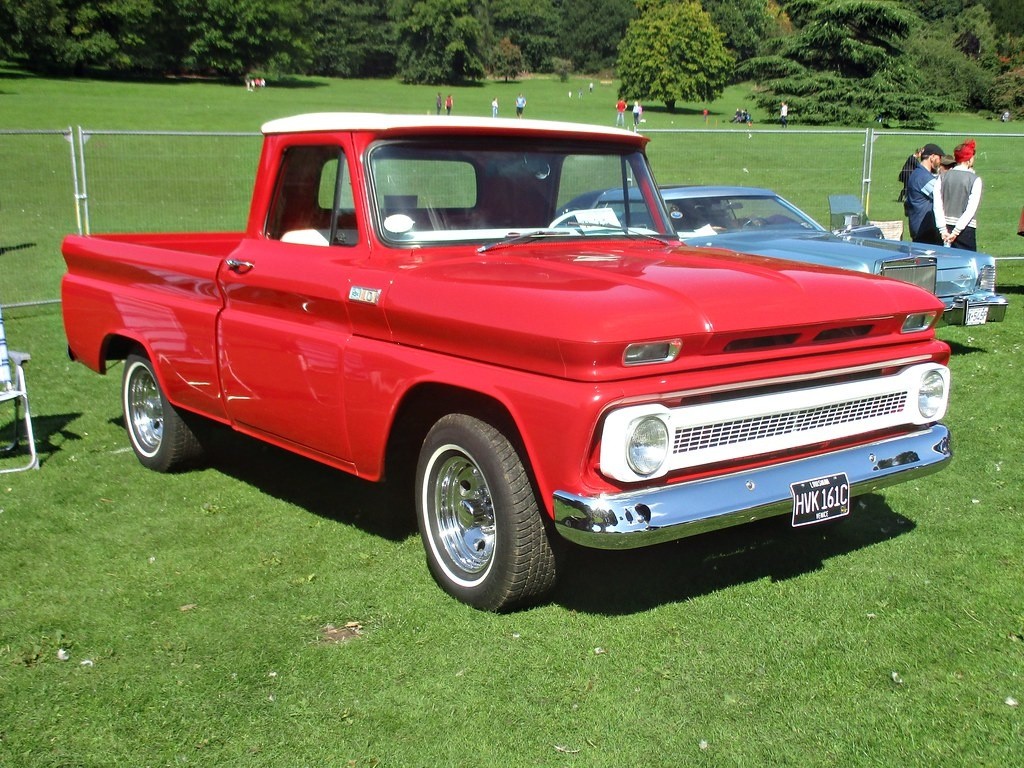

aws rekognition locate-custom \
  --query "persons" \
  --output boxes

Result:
[244,74,267,93]
[611,93,791,131]
[430,76,597,118]
[896,140,985,252]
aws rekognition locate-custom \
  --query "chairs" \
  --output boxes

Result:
[0,305,41,474]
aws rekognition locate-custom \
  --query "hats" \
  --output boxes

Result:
[921,144,945,155]
[941,154,957,165]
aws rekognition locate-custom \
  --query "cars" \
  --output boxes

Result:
[555,184,1009,329]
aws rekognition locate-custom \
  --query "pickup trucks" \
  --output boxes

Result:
[61,111,955,617]
